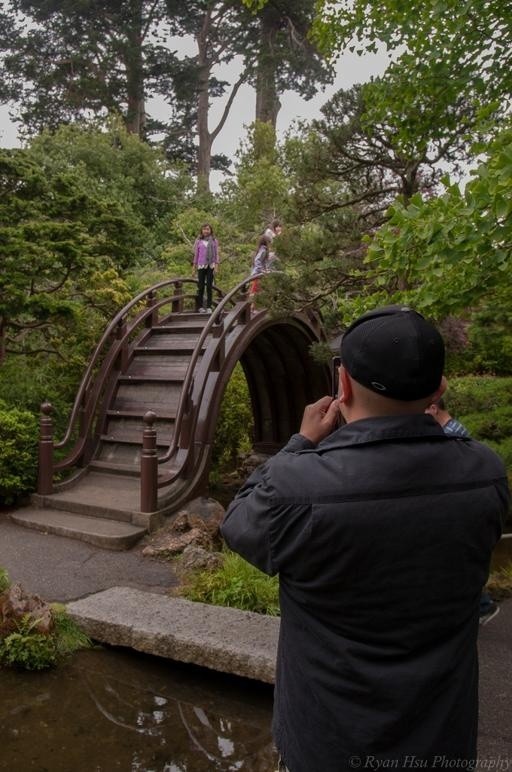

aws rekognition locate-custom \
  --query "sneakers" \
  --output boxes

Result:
[196,307,213,313]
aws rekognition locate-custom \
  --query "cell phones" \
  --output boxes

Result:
[333,357,347,428]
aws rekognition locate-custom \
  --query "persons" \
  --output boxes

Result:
[253,223,281,273]
[424,394,501,628]
[192,224,219,312]
[219,304,511,772]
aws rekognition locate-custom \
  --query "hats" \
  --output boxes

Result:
[338,303,447,404]
